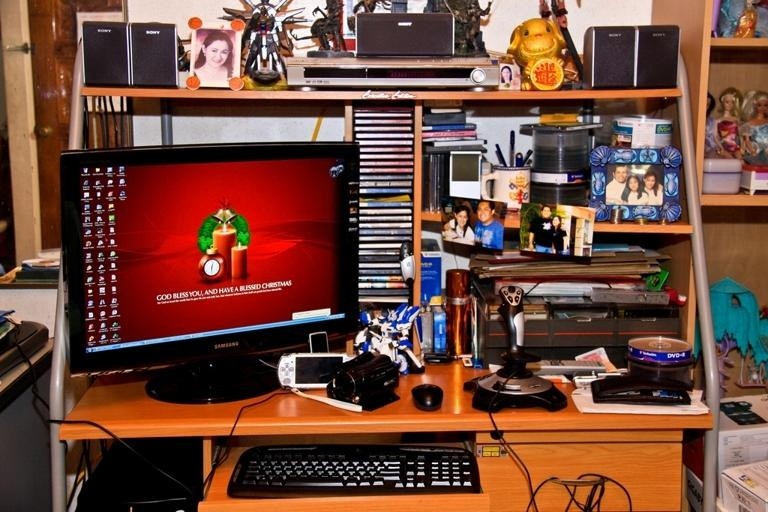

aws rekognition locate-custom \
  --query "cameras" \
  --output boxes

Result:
[326,351,401,412]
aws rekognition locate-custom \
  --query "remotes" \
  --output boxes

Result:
[525,359,606,374]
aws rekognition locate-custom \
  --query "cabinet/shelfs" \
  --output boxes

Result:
[648,0,767,389]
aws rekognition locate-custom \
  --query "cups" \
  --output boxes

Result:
[479,163,532,209]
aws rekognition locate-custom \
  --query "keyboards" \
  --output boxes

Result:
[227,443,483,499]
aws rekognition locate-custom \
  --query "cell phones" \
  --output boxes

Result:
[308,331,330,352]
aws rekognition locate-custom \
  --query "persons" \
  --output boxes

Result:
[501,64,514,84]
[704,94,722,158]
[474,201,503,249]
[622,175,647,206]
[528,206,553,252]
[550,216,568,254]
[644,173,663,204]
[605,165,629,203]
[743,90,768,165]
[194,31,234,84]
[441,206,474,243]
[713,87,742,159]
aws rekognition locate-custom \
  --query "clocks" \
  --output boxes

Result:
[198,248,225,282]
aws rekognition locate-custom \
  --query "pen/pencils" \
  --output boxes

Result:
[516,153,523,167]
[495,144,508,167]
[523,150,533,165]
[510,130,515,167]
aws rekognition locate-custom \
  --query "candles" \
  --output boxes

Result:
[213,222,236,274]
[231,241,248,278]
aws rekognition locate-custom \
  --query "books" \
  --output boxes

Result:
[427,155,444,212]
[422,107,487,152]
[468,245,673,319]
[351,106,412,300]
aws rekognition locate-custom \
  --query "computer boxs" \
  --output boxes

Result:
[76,436,201,511]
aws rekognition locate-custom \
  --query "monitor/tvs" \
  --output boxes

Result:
[59,141,360,405]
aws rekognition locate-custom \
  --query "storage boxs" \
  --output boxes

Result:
[701,394,768,498]
[717,458,768,512]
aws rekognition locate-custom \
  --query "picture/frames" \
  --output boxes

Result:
[587,144,683,223]
[184,15,248,94]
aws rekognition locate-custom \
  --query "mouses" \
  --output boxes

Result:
[411,383,444,412]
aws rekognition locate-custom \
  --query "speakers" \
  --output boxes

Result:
[353,12,455,57]
[582,25,636,89]
[129,22,179,88]
[82,20,130,87]
[637,26,681,89]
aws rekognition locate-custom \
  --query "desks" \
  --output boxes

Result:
[49,39,719,511]
